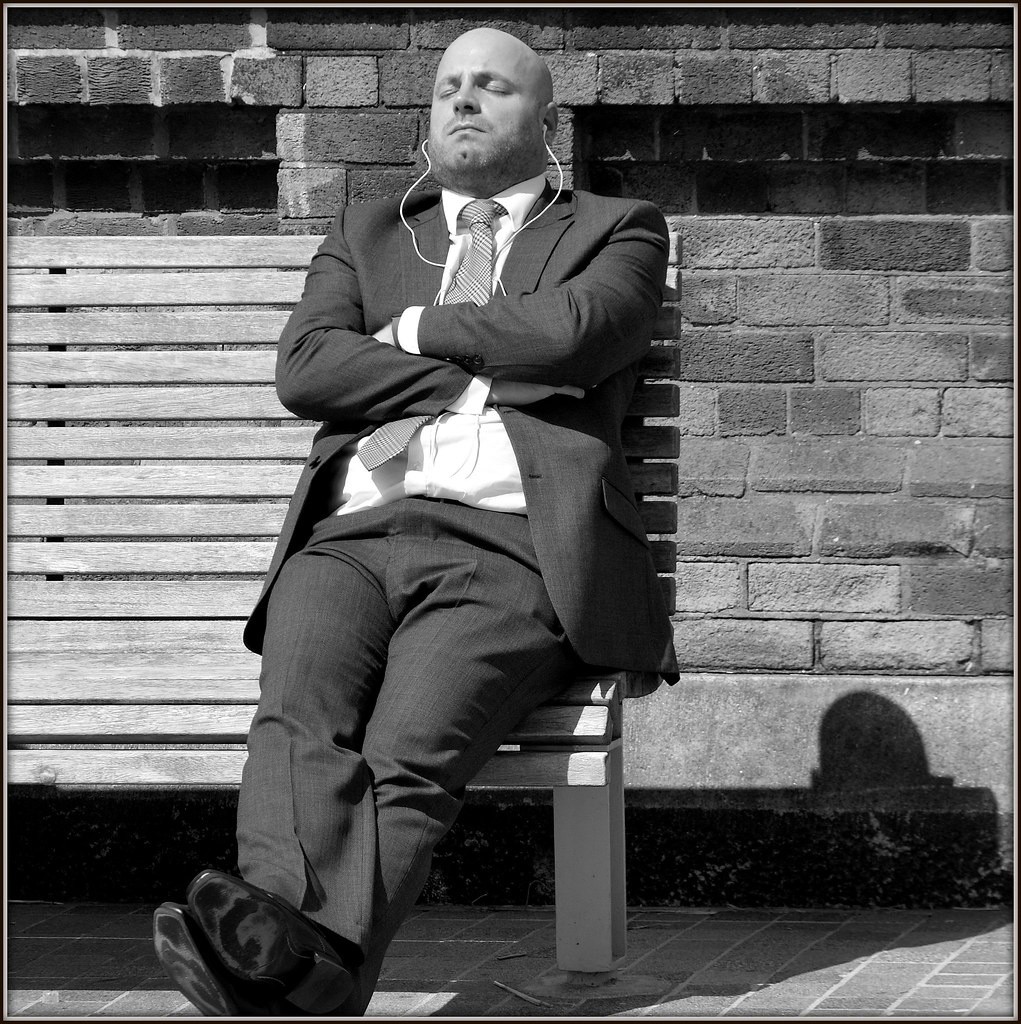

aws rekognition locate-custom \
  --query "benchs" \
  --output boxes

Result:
[7,229,683,988]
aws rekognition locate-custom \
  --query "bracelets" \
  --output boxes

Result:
[390,314,403,350]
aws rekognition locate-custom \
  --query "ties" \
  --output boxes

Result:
[358,197,503,470]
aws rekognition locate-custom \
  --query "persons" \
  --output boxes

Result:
[152,29,681,1018]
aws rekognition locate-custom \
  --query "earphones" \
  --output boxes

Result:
[542,124,547,133]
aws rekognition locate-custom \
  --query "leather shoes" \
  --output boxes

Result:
[153,902,241,1019]
[187,874,351,1014]
